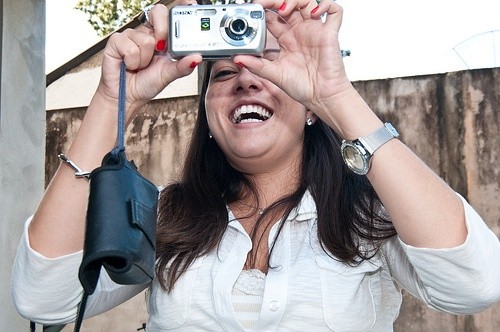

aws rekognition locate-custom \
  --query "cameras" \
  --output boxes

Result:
[169,3,266,61]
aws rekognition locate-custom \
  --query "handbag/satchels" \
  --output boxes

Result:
[79,60,159,295]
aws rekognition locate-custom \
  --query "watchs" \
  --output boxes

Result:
[332,122,400,178]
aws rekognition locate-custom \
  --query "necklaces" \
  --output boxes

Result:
[235,197,297,215]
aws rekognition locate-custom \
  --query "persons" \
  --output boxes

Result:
[15,1,500,331]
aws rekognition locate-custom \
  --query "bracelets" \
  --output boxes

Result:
[56,151,92,184]
